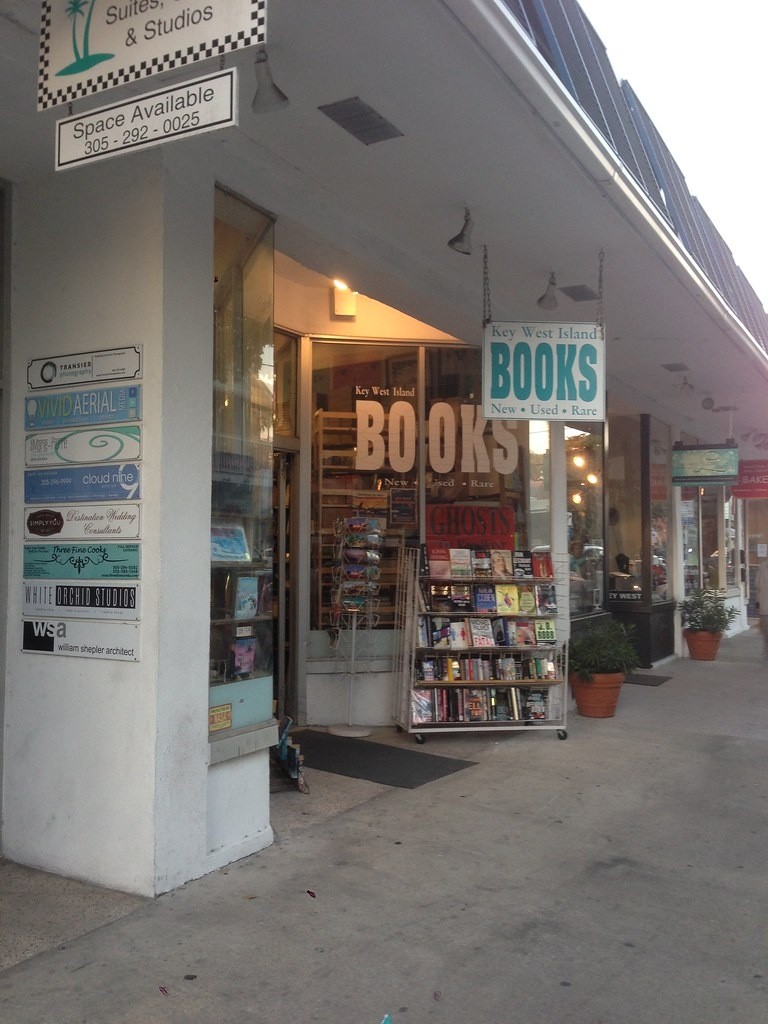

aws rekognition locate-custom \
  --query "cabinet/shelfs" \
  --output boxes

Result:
[324,516,383,737]
[395,542,572,743]
[313,407,524,629]
[212,446,271,685]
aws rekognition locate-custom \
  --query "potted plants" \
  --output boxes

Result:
[678,587,742,662]
[568,619,642,719]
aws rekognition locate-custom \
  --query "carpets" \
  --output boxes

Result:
[624,670,671,687]
[286,728,480,790]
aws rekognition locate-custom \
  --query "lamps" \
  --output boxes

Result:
[448,206,474,256]
[740,429,755,442]
[702,394,714,409]
[756,435,768,451]
[253,44,289,113]
[535,276,559,310]
